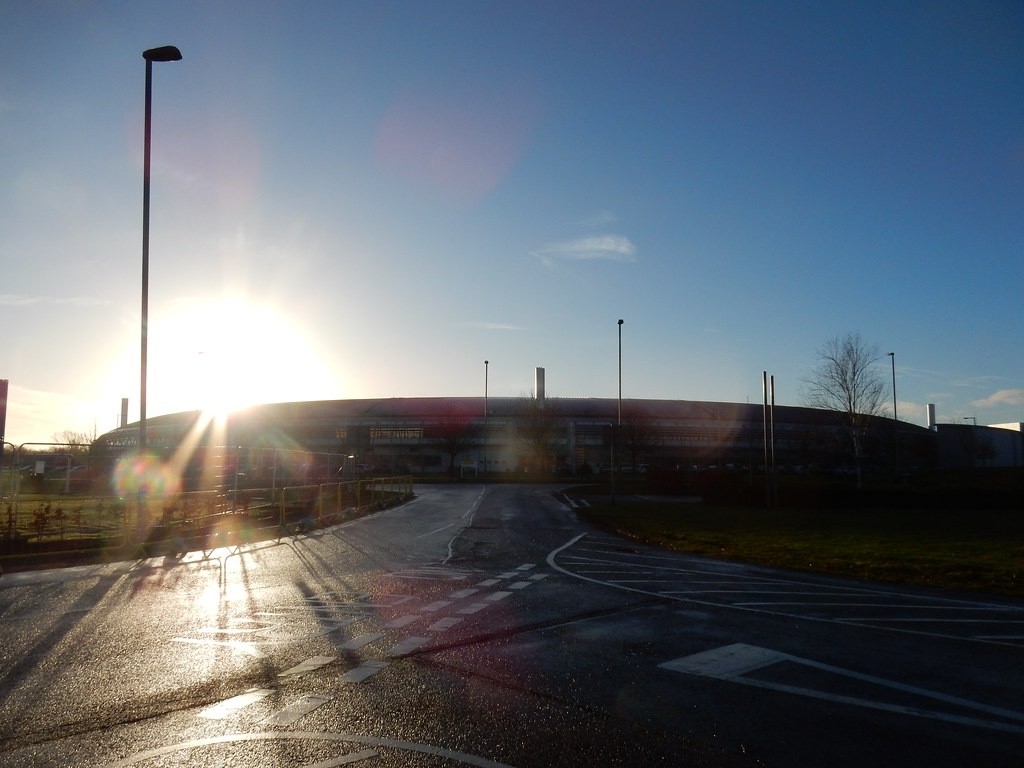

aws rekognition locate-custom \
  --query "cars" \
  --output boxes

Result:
[600,464,816,473]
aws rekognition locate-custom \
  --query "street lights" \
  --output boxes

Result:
[134,45,185,542]
[887,352,897,419]
[484,360,489,484]
[617,319,624,426]
[964,417,977,425]
